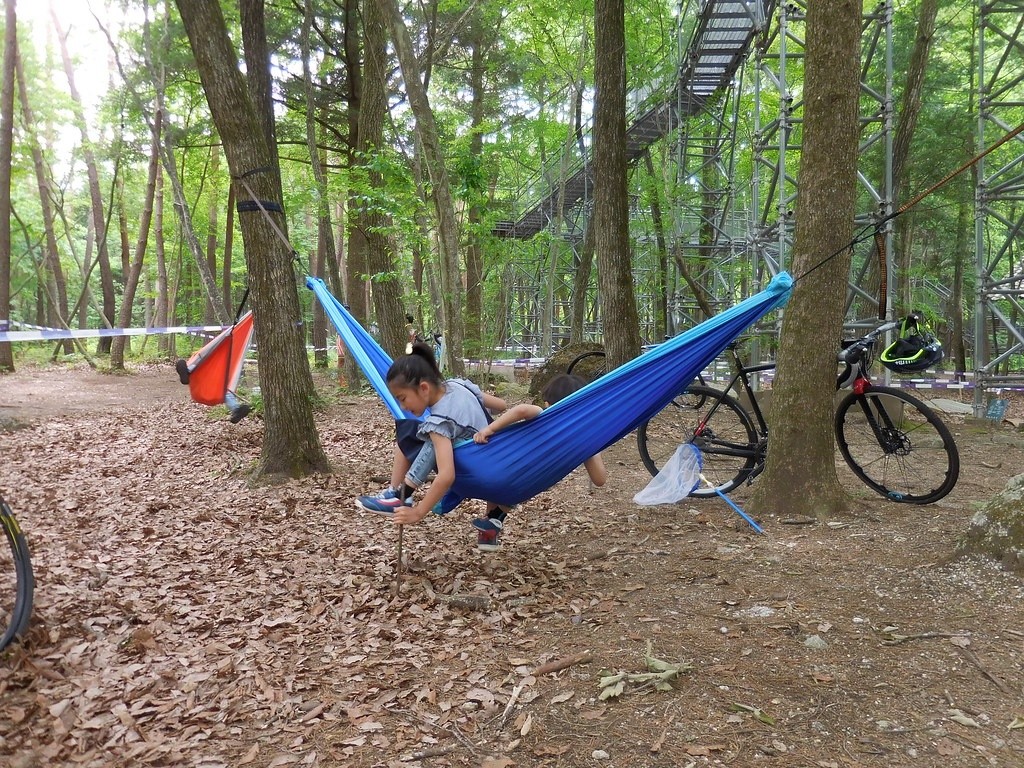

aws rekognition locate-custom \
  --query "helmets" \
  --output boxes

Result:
[881,331,945,373]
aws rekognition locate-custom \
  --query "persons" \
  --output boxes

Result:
[471,372,607,536]
[176,360,251,425]
[404,314,418,343]
[353,342,506,553]
[336,304,350,369]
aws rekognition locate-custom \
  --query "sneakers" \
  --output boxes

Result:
[471,518,502,538]
[477,530,499,552]
[354,485,414,518]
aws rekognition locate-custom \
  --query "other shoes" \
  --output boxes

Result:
[176,359,190,385]
[230,404,250,424]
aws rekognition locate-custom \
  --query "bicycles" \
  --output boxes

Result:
[636,314,960,503]
[566,334,707,410]
